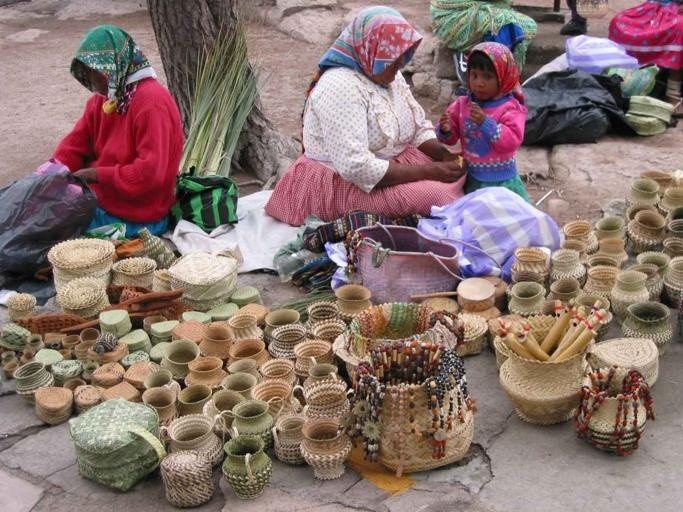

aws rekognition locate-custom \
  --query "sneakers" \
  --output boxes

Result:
[454,51,467,84]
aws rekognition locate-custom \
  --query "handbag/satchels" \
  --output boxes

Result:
[417,187,567,280]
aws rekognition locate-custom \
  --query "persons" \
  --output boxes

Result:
[50,25,185,242]
[432,42,530,205]
[428,0,538,75]
[261,4,469,227]
